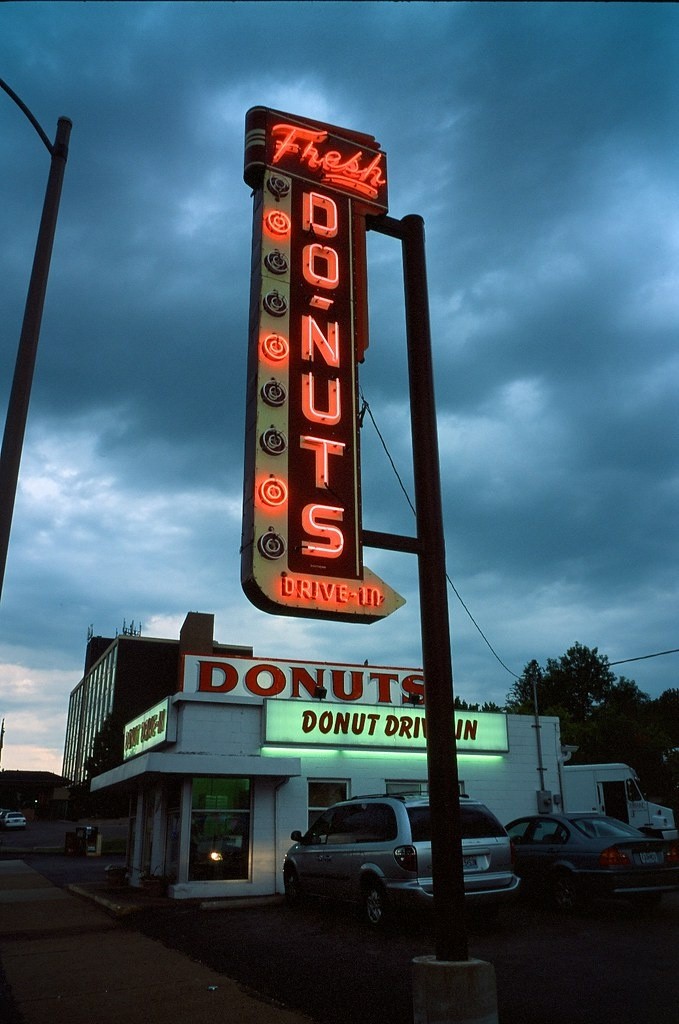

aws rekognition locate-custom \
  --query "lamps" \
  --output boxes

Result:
[315,686,327,700]
[410,694,426,706]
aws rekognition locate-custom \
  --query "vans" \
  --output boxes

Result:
[284,796,519,920]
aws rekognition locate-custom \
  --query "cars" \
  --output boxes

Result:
[6,811,29,828]
[509,815,673,917]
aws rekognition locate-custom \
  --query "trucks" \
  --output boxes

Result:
[560,762,678,848]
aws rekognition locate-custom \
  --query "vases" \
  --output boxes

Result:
[142,879,168,897]
[108,868,128,888]
